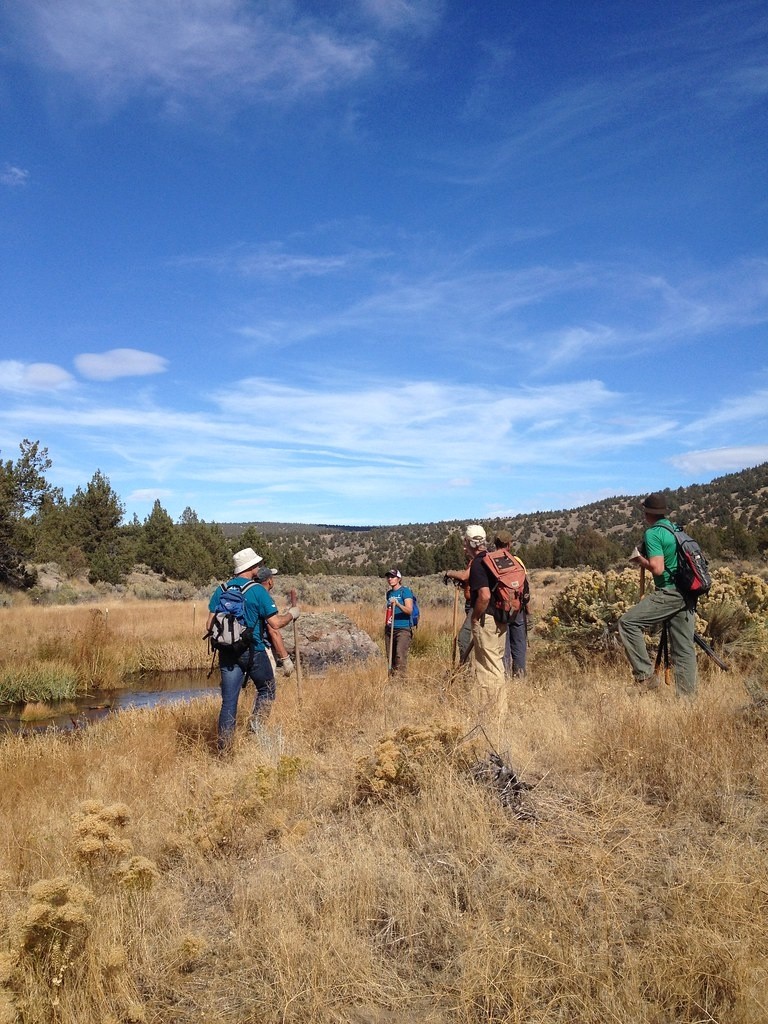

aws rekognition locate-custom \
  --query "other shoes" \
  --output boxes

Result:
[628,677,657,695]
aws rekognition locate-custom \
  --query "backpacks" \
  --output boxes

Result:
[203,579,258,656]
[647,522,712,596]
[401,585,420,628]
[476,547,528,619]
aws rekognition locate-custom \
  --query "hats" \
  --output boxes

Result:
[385,568,401,578]
[465,524,485,545]
[254,567,277,583]
[494,531,514,543]
[233,547,263,574]
[637,493,669,514]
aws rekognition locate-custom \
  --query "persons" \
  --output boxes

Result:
[618,492,697,697]
[385,569,413,677]
[443,525,528,715]
[206,548,294,752]
[253,567,294,678]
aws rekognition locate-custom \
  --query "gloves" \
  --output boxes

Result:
[288,607,299,620]
[281,655,294,677]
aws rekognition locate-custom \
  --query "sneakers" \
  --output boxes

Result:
[248,718,265,733]
[217,740,236,756]
